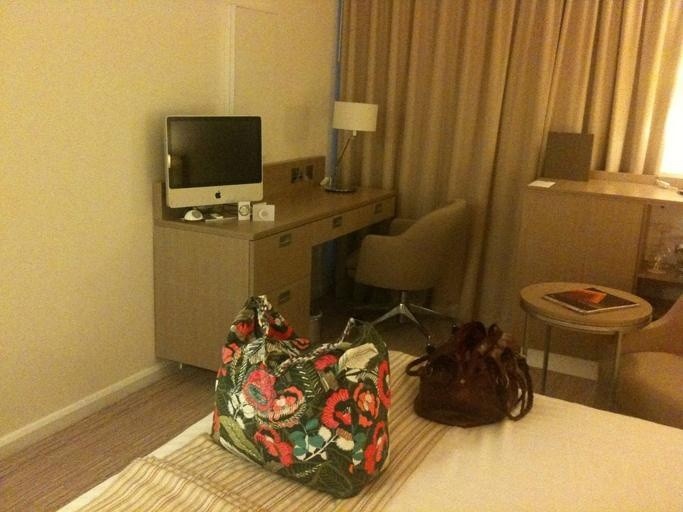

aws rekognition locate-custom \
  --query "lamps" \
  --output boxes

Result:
[320,100,379,194]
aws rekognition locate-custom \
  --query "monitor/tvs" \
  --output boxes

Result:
[163,114,265,222]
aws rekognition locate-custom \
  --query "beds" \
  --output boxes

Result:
[59,350,680,510]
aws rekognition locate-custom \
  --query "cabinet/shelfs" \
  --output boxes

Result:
[512,171,683,296]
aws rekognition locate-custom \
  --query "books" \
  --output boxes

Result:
[541,288,639,316]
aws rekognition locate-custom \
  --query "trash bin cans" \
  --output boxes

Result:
[310,308,324,346]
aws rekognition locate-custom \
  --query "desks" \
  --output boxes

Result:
[153,157,396,373]
[519,283,653,394]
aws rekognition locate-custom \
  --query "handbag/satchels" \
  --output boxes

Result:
[211,296,389,500]
[407,320,533,427]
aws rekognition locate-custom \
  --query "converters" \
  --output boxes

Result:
[658,180,670,189]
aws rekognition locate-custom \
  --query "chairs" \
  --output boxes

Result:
[354,200,470,352]
